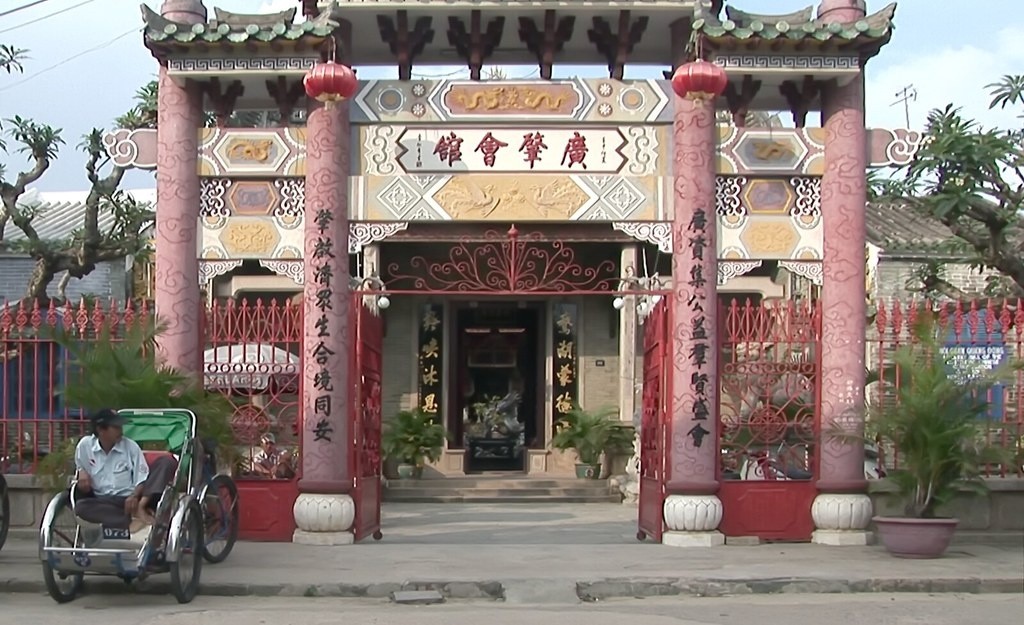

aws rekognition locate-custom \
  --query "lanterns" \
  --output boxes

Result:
[670,58,727,110]
[303,60,358,111]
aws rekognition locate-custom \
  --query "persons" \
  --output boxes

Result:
[253,432,295,480]
[73,408,176,533]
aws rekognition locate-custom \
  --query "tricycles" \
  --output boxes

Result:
[37,406,242,605]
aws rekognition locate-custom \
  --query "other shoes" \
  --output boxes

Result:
[133,511,155,525]
[128,519,146,533]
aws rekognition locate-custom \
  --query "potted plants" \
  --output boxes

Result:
[805,298,1024,559]
[378,408,454,479]
[544,401,635,479]
[467,393,517,459]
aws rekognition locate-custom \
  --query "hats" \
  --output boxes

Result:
[260,432,276,445]
[91,409,133,425]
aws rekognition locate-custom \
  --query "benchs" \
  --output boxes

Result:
[141,451,178,515]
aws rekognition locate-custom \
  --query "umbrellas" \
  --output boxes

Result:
[204,342,299,472]
